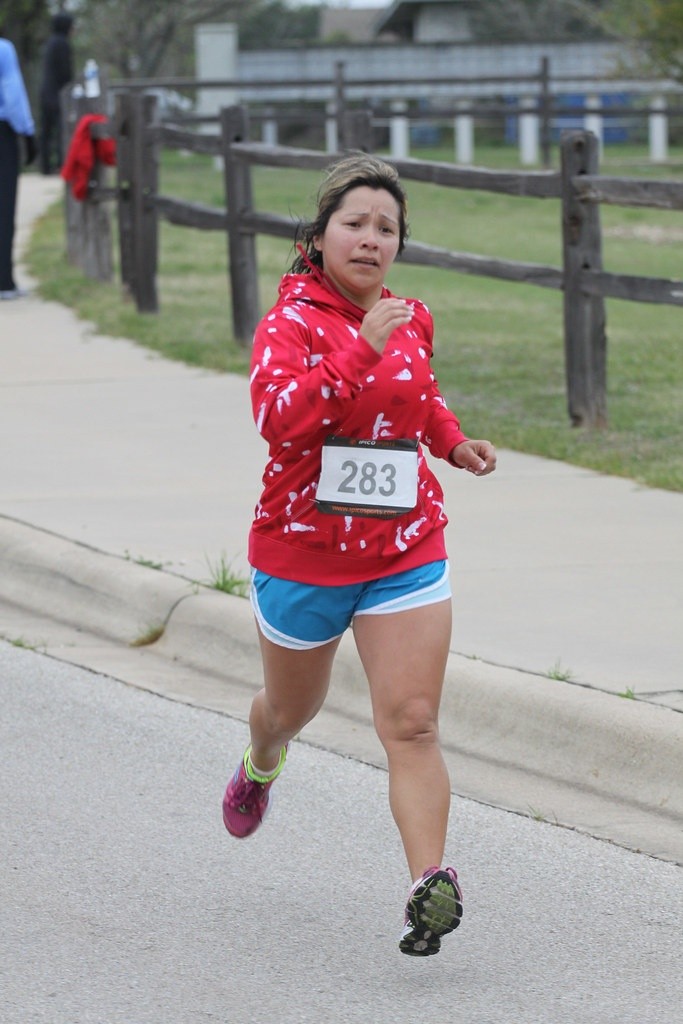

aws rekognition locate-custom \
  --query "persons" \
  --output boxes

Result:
[0,36,38,302]
[39,11,76,175]
[220,157,498,957]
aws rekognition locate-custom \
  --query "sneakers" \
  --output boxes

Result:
[221,738,291,840]
[398,866,463,960]
